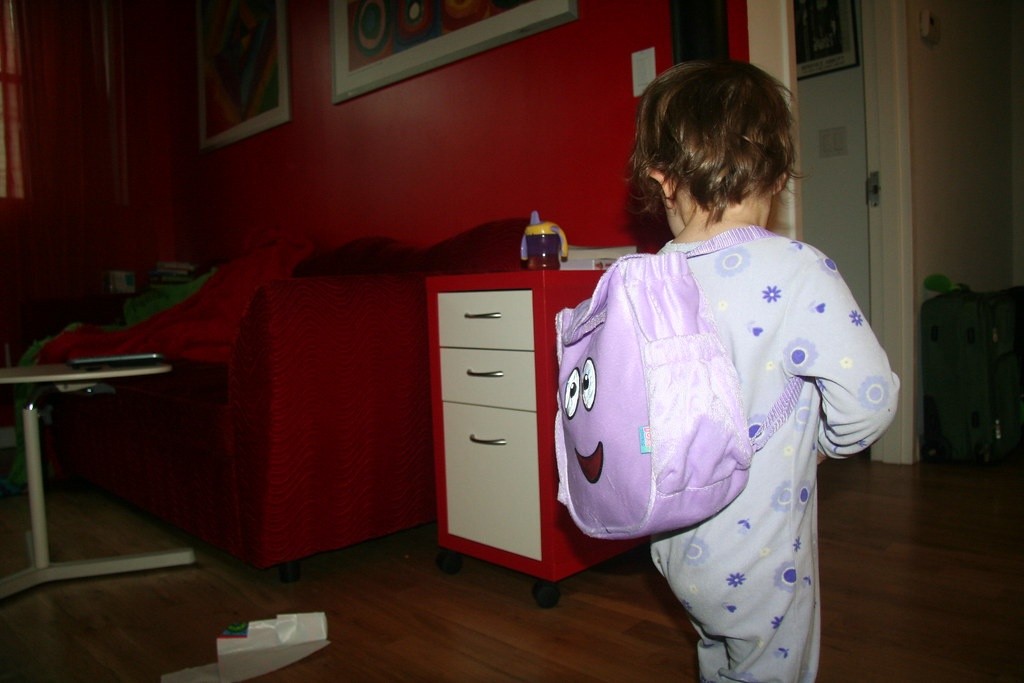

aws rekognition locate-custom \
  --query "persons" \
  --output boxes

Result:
[629,53,901,683]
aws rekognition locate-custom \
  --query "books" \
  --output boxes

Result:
[145,260,198,291]
[558,244,639,270]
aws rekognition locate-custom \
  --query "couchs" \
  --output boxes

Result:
[23,218,538,588]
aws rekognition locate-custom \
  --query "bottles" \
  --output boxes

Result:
[520,211,569,271]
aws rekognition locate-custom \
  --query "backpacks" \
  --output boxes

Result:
[555,225,805,538]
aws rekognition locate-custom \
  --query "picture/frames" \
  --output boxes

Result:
[793,0,860,83]
[195,0,293,153]
[329,0,578,108]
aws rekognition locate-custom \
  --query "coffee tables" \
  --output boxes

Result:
[0,364,199,603]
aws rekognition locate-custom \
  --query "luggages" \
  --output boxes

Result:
[919,283,1022,465]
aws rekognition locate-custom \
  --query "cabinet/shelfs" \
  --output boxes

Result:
[425,268,653,607]
